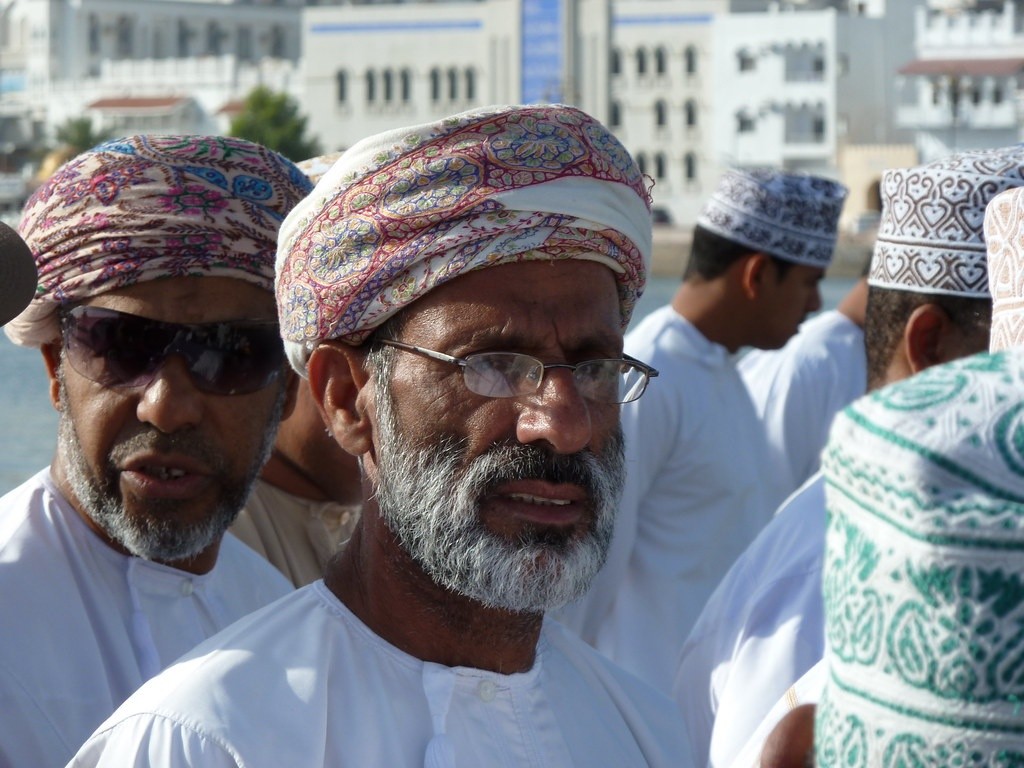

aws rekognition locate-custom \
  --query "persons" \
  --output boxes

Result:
[67,102,691,768]
[544,152,849,698]
[0,134,317,768]
[730,173,883,487]
[228,153,361,591]
[670,144,1024,768]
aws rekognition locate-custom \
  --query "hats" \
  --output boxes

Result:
[983,185,1024,353]
[812,352,1024,767]
[868,147,1024,298]
[1,134,316,348]
[695,164,849,266]
[273,103,654,382]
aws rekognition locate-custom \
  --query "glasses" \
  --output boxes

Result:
[59,304,283,396]
[358,337,659,403]
